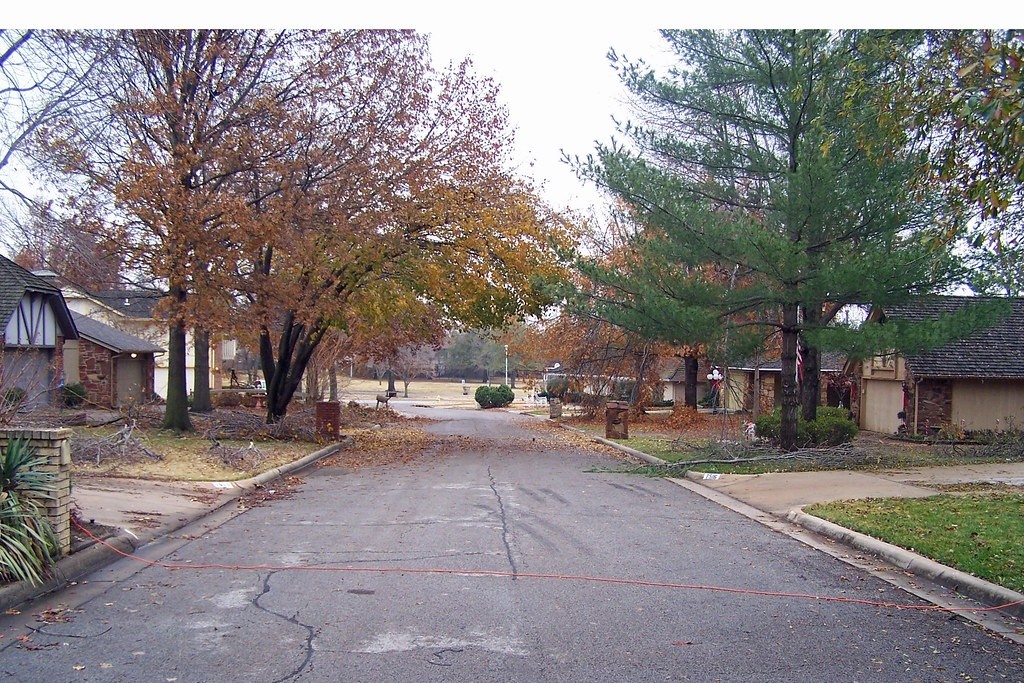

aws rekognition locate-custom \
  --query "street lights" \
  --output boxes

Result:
[504,344,509,387]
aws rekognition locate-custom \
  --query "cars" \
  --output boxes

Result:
[251,379,267,395]
